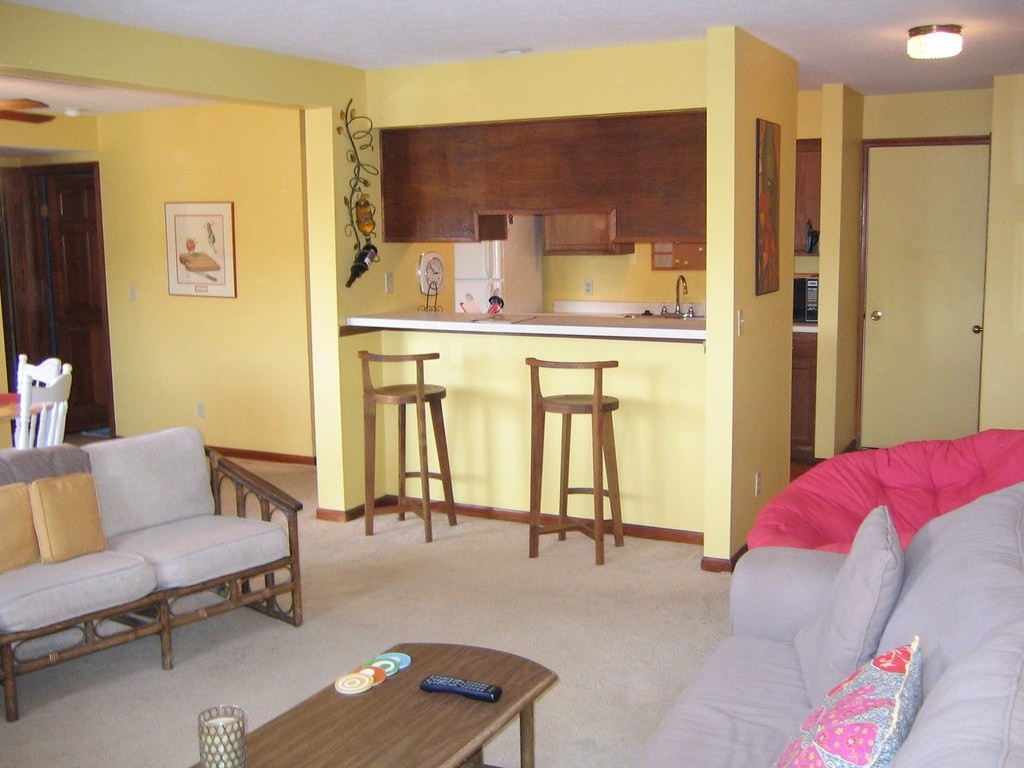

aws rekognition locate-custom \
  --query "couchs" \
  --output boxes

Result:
[636,427,1024,768]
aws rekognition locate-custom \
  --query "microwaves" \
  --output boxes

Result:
[787,273,818,326]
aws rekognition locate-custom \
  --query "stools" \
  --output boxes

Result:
[525,356,625,566]
[356,351,457,543]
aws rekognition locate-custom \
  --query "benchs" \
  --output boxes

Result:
[0,425,303,723]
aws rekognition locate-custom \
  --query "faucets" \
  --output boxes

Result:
[674,274,689,314]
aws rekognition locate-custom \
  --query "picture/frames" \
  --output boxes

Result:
[164,201,237,297]
[754,117,781,295]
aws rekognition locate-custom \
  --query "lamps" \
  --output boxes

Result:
[908,24,964,59]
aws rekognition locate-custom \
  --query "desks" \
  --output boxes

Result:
[188,642,560,768]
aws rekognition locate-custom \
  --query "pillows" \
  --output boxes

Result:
[1,482,42,573]
[27,472,108,565]
[763,634,922,768]
[790,504,908,706]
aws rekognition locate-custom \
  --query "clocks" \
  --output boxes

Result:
[418,252,445,312]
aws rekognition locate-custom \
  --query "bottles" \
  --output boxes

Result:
[356,199,374,246]
[346,245,378,288]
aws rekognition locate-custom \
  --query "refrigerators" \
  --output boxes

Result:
[453,214,543,314]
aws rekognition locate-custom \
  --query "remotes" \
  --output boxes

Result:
[420,674,503,703]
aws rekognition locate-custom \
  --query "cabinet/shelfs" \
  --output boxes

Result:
[379,107,707,269]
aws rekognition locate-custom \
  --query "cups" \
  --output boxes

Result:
[197,705,247,768]
